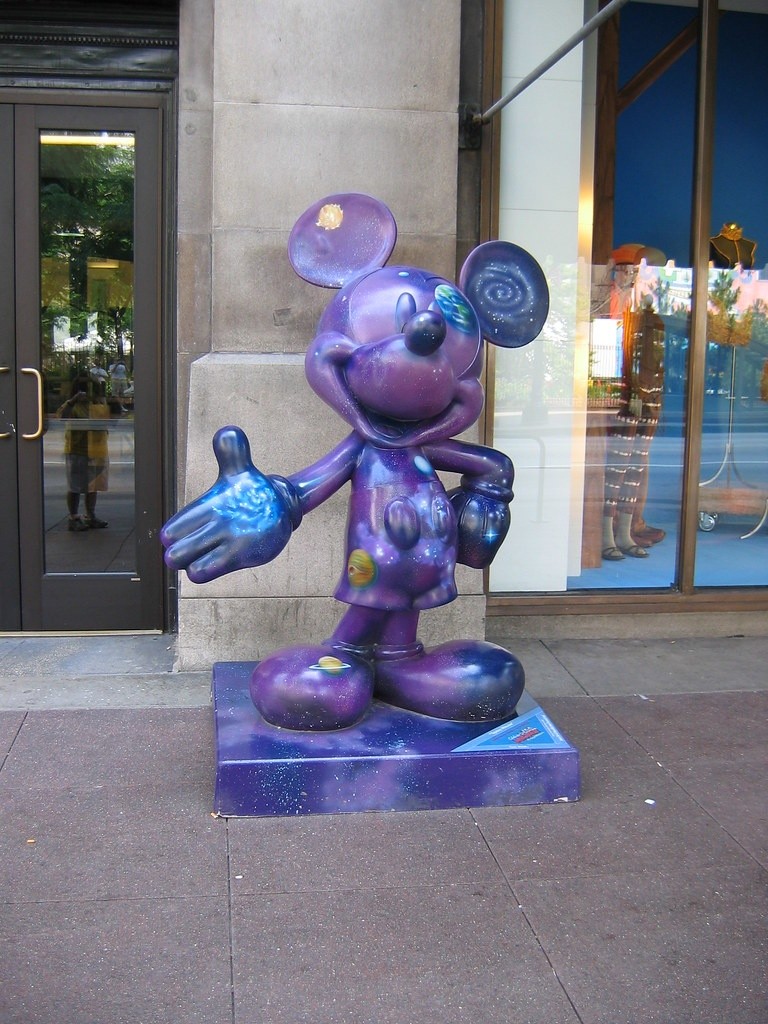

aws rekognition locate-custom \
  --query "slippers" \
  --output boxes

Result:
[616,545,650,558]
[602,547,625,559]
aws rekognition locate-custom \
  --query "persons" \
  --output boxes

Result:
[70,354,129,412]
[56,370,110,531]
[603,248,667,560]
[707,223,756,346]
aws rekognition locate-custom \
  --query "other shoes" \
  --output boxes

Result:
[631,526,666,543]
[615,530,652,546]
[122,408,129,412]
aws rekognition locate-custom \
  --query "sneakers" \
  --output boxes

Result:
[68,518,89,531]
[84,516,109,528]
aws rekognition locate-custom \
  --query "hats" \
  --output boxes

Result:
[612,244,645,266]
